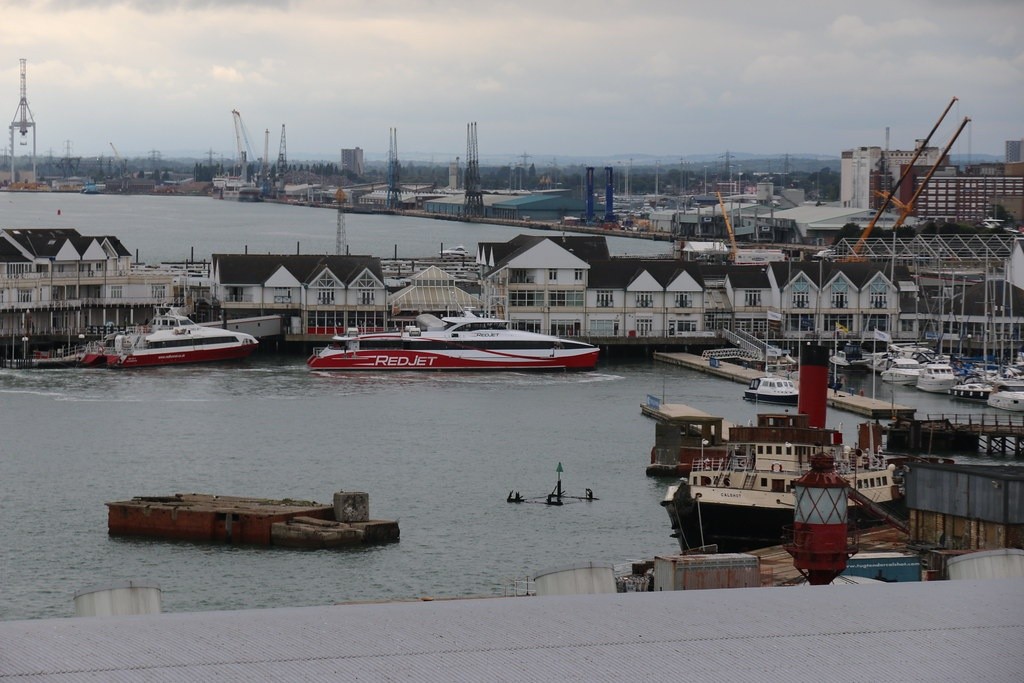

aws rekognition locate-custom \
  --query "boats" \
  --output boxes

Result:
[3,314,259,368]
[306,309,600,371]
[743,377,798,406]
[660,410,900,554]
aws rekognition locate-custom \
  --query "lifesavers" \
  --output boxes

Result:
[771,462,782,471]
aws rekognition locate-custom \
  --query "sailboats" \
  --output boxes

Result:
[831,232,1024,410]
